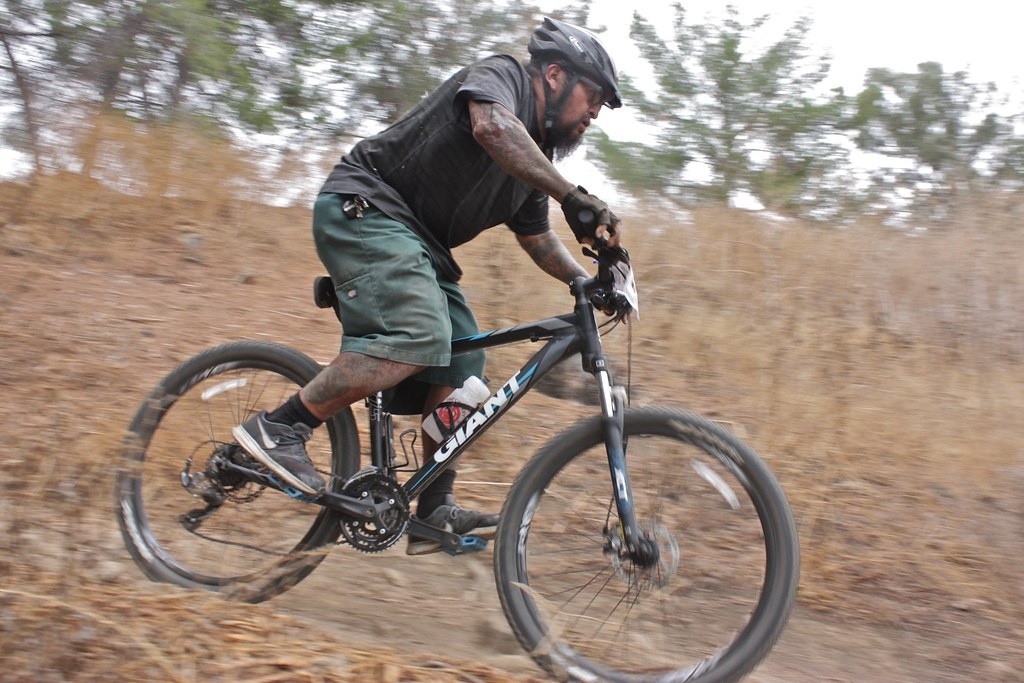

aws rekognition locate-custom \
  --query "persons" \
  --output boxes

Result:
[230,17,623,555]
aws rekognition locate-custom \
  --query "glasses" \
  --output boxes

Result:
[561,67,605,105]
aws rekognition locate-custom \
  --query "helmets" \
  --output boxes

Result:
[527,17,623,107]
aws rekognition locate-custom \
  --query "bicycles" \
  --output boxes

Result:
[111,207,803,683]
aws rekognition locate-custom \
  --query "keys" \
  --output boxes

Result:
[341,192,369,220]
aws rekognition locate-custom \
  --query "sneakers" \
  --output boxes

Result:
[405,494,500,556]
[232,410,327,497]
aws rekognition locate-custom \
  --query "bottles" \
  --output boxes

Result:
[422,374,491,444]
